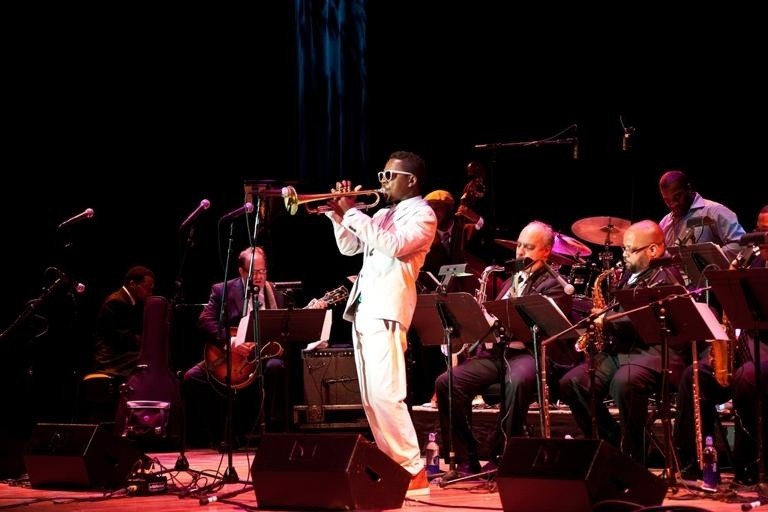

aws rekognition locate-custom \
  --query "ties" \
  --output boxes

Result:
[506,275,523,297]
[442,231,450,249]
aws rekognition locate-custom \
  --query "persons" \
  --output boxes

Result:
[96,266,156,434]
[199,245,294,453]
[331,151,438,495]
[423,171,768,487]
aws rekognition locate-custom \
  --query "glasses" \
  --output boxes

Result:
[376,169,414,181]
[622,245,650,254]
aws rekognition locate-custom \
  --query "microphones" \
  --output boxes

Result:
[177,197,212,228]
[254,191,265,232]
[503,259,524,271]
[621,133,633,151]
[62,207,96,228]
[740,232,765,245]
[648,255,673,267]
[228,200,255,217]
[573,126,580,158]
[52,266,86,293]
[541,260,576,297]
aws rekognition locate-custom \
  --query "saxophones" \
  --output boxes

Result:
[704,244,765,388]
[440,264,505,358]
[575,260,622,360]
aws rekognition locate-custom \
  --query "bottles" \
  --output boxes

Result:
[426,433,440,474]
[702,435,718,489]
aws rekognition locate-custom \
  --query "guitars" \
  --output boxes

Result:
[204,285,349,391]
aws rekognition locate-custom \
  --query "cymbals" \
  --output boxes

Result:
[494,238,576,267]
[551,231,592,257]
[570,216,632,246]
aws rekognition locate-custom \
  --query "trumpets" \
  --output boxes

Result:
[283,185,391,217]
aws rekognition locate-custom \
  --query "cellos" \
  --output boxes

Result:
[434,160,482,298]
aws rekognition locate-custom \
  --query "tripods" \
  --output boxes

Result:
[606,287,718,494]
[427,329,479,481]
[149,228,224,487]
[439,274,551,488]
[198,204,267,506]
[178,218,253,499]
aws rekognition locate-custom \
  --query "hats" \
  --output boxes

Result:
[423,189,454,206]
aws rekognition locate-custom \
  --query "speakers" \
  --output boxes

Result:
[496,435,669,512]
[23,420,127,489]
[250,430,413,512]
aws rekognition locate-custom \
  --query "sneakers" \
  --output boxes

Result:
[215,435,238,450]
[407,469,433,496]
[681,453,705,479]
